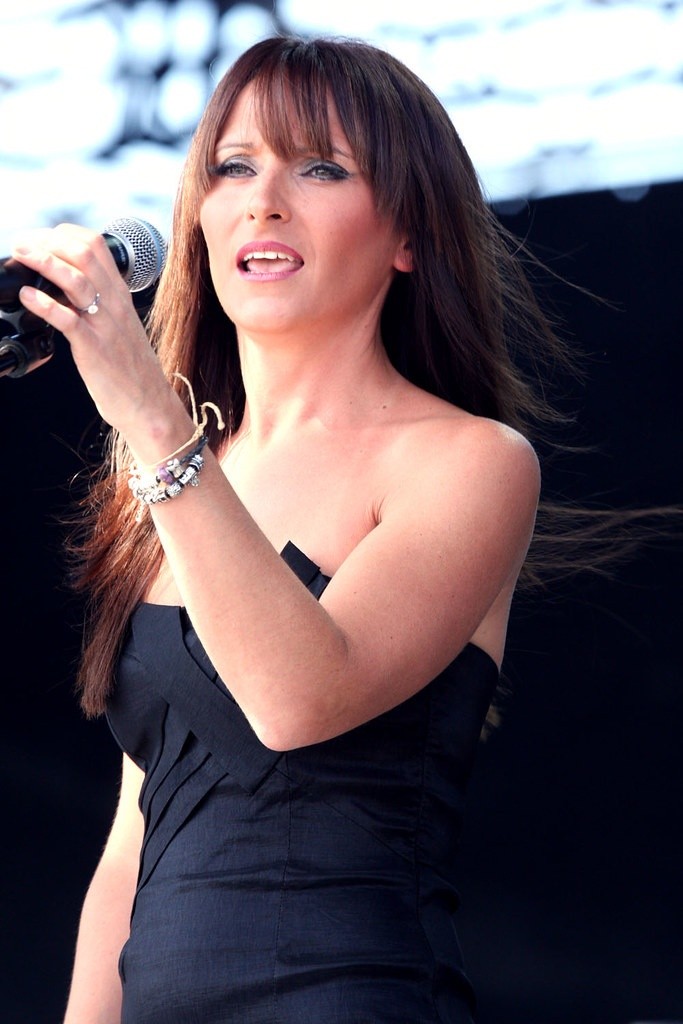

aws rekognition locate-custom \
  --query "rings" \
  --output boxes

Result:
[78,292,103,315]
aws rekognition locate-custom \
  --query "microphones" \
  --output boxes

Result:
[0,216,167,313]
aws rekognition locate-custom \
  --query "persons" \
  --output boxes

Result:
[17,37,541,1023]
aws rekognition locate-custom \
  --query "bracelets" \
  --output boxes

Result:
[109,370,226,475]
[127,435,209,508]
